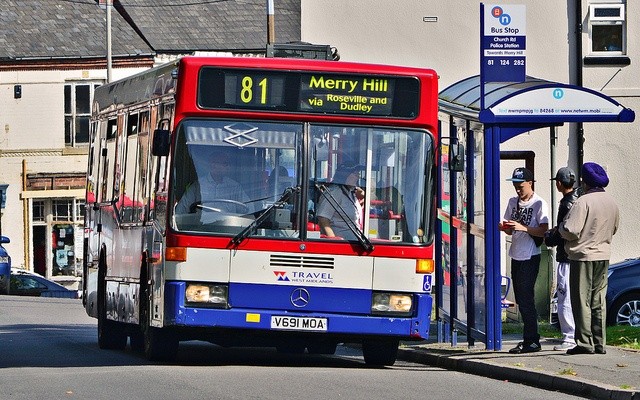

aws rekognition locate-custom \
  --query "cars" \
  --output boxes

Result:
[606,255,640,328]
[9,274,79,300]
[0,236,12,294]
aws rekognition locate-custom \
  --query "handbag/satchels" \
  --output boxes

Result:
[517,197,544,247]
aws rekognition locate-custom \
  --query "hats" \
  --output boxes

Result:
[506,168,536,182]
[550,167,576,183]
[581,162,609,187]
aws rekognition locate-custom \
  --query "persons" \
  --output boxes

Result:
[500,167,549,355]
[87,163,126,206]
[176,152,254,214]
[560,162,619,354]
[546,167,578,351]
[316,161,365,239]
[267,165,289,198]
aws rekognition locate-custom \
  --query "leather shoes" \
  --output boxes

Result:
[567,345,596,354]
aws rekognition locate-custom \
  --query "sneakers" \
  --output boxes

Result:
[554,342,574,351]
[509,343,530,353]
[528,342,538,352]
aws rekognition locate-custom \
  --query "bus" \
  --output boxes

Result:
[82,57,439,366]
